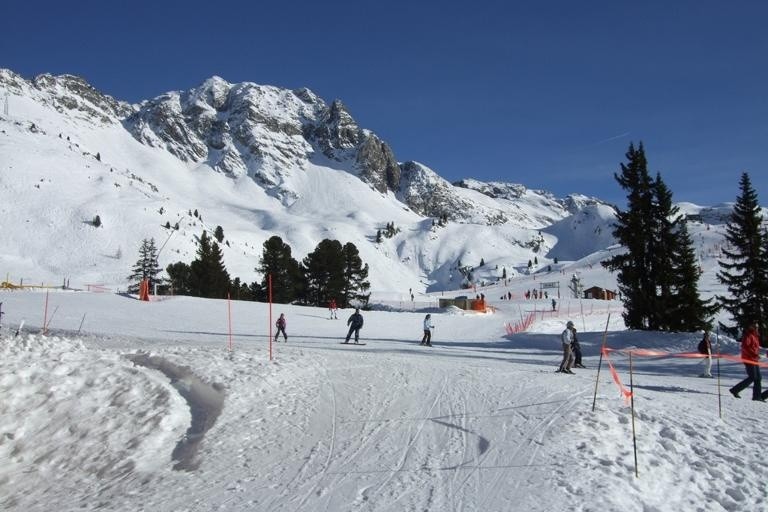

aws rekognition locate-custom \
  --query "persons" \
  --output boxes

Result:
[274,312,288,342]
[475,289,557,312]
[329,298,338,320]
[698,319,768,402]
[344,308,363,343]
[559,320,576,375]
[570,327,586,369]
[419,314,435,347]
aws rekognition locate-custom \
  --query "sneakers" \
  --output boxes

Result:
[562,363,586,373]
[729,389,741,398]
